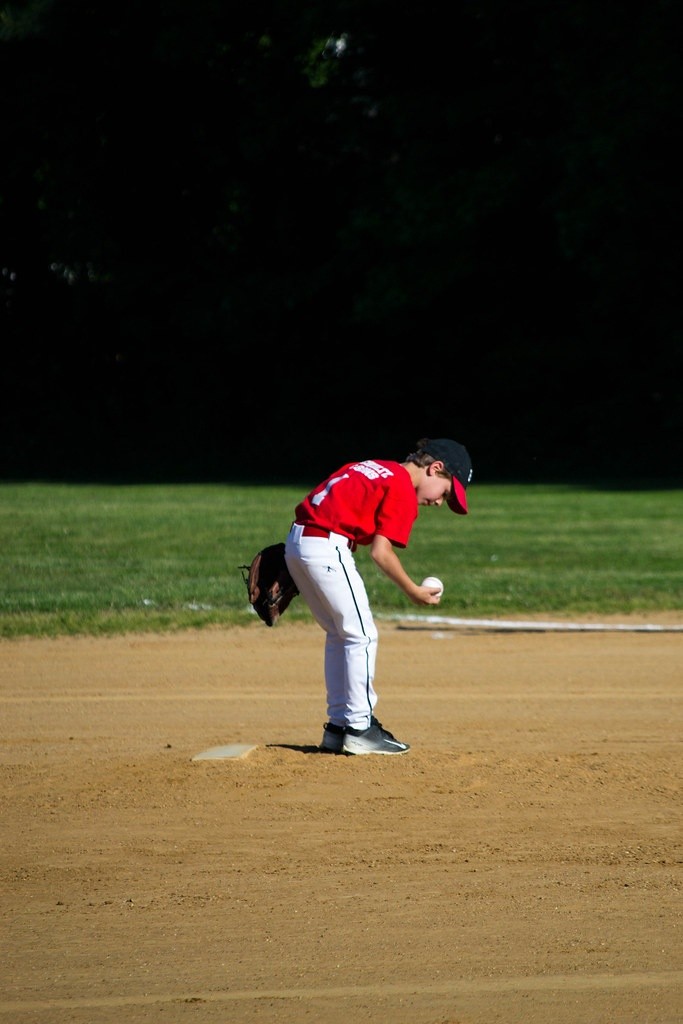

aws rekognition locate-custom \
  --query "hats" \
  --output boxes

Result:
[422,438,473,514]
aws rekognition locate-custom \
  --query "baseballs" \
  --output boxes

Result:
[421,575,445,600]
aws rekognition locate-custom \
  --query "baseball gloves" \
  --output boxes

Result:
[243,539,300,625]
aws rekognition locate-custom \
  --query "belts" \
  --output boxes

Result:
[290,524,351,549]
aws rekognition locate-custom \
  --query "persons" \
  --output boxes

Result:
[284,438,473,755]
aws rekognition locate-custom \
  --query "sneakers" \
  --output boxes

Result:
[321,722,341,749]
[341,722,410,755]
